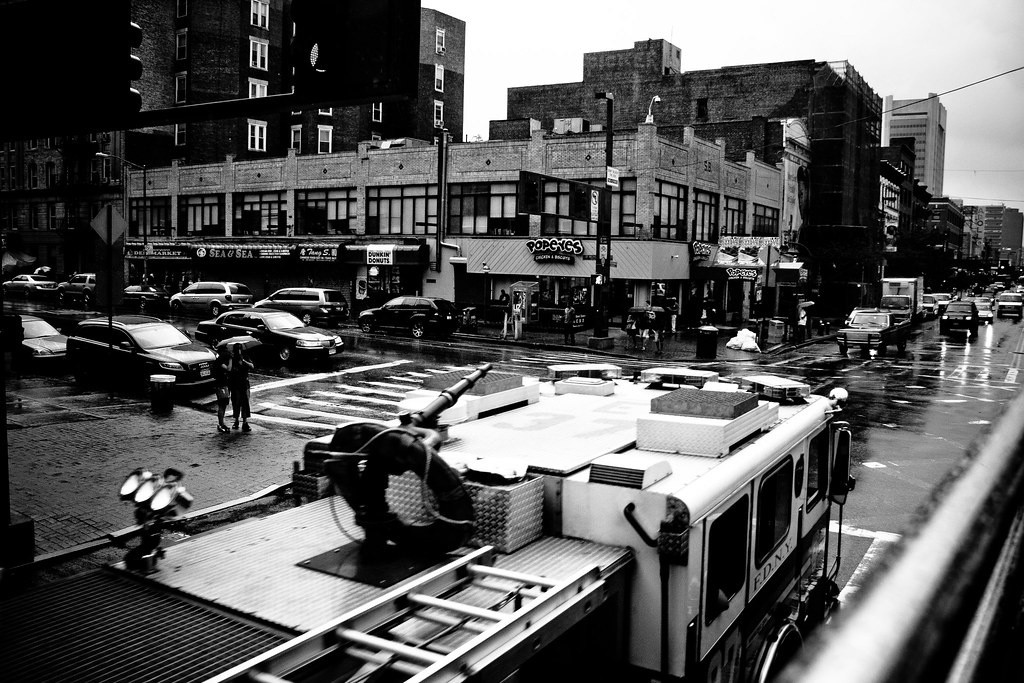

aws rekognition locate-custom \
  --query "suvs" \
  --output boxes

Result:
[355,294,458,340]
[940,301,980,336]
[57,273,99,302]
[252,287,351,327]
[167,280,253,314]
[844,306,893,330]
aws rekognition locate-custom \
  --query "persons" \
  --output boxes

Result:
[500,289,510,301]
[625,315,665,352]
[212,343,254,433]
[564,300,576,345]
[670,297,679,333]
[646,301,651,307]
[798,307,808,344]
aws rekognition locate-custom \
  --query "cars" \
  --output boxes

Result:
[935,293,953,310]
[922,295,940,317]
[2,274,59,297]
[962,278,1024,324]
[124,284,166,308]
[1,315,69,362]
[66,314,223,398]
[997,292,1024,317]
[198,308,344,363]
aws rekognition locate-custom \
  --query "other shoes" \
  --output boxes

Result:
[217,424,229,432]
[242,423,251,430]
[233,420,238,429]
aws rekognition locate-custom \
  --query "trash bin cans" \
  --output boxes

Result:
[463,306,478,333]
[694,326,719,359]
[768,319,784,344]
[150,375,176,417]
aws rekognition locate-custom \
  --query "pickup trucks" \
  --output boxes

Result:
[834,309,913,357]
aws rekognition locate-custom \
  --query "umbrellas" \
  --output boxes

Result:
[797,301,814,308]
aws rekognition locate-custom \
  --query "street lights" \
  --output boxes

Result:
[93,151,151,284]
[588,92,619,350]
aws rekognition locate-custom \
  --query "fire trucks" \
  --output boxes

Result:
[0,348,870,683]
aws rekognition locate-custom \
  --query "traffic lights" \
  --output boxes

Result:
[289,2,348,104]
[74,1,145,127]
[590,273,606,287]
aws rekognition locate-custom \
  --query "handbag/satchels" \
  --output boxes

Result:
[215,387,229,401]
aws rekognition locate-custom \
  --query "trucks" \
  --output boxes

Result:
[879,276,925,315]
